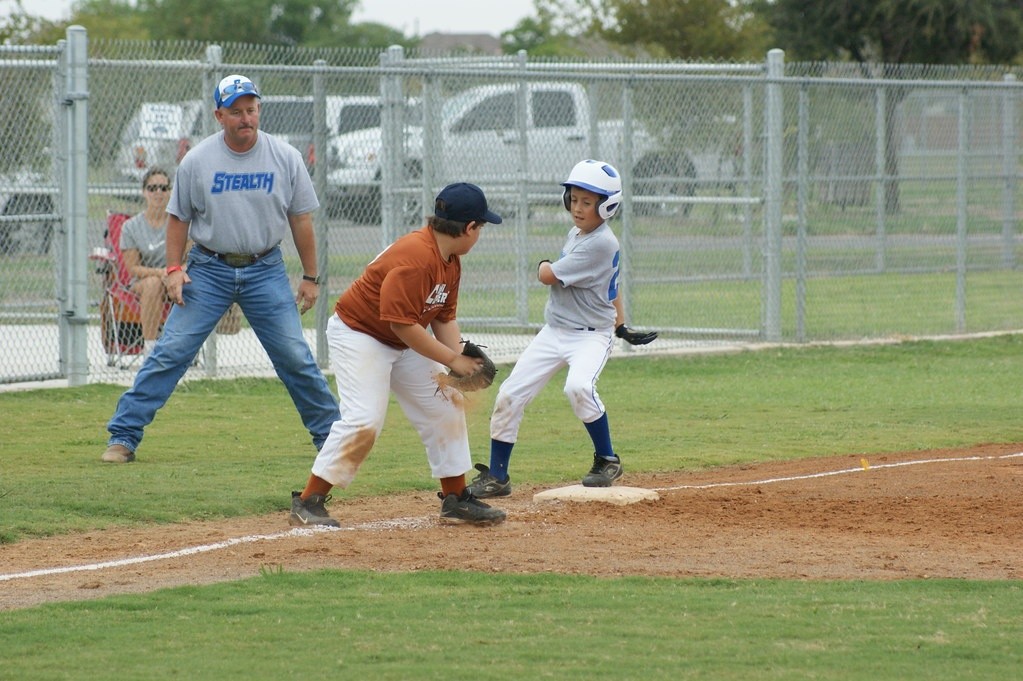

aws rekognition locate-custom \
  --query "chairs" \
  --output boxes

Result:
[90,210,206,369]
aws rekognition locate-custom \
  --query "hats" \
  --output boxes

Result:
[435,182,503,224]
[213,73,262,109]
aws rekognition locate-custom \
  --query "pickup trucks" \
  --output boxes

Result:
[333,79,744,228]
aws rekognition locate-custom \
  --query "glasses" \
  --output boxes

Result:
[146,184,169,192]
[216,81,258,108]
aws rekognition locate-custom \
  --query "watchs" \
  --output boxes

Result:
[302,275,321,284]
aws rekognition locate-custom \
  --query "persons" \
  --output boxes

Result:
[118,169,194,365]
[466,159,658,500]
[100,75,340,464]
[286,183,507,529]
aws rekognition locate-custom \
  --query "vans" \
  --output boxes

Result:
[117,90,426,182]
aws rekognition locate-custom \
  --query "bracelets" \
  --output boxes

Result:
[167,265,181,275]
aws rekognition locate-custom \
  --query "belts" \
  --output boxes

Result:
[192,241,279,267]
[574,327,595,331]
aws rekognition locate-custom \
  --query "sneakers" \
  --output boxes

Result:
[582,451,624,486]
[437,486,506,525]
[288,491,340,529]
[467,463,513,498]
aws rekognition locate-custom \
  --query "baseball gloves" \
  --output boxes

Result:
[440,340,496,393]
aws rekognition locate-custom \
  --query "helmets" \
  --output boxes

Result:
[615,323,658,345]
[560,158,622,220]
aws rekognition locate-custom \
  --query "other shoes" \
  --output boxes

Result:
[102,444,135,464]
